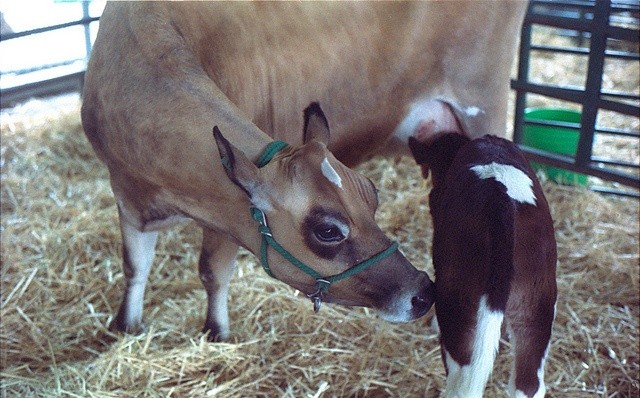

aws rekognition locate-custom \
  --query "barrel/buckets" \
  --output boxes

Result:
[521,108,589,187]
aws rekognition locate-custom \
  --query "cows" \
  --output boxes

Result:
[80,0,531,343]
[407,129,558,397]
